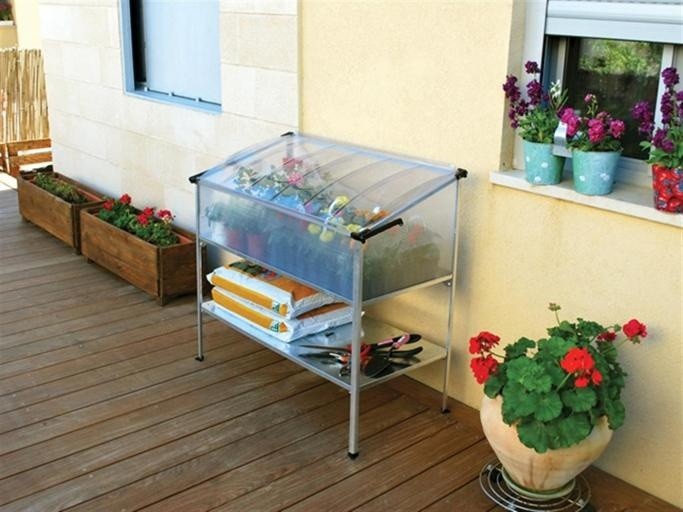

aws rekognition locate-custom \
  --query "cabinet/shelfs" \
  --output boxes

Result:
[186,127,468,460]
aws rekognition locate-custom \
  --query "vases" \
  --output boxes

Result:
[522,136,683,214]
[478,390,616,497]
[79,204,208,307]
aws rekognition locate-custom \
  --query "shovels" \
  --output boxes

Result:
[365,336,408,378]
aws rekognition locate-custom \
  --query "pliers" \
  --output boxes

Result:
[370,335,423,357]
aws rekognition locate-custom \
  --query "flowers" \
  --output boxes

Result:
[461,298,655,454]
[99,192,179,245]
[501,49,683,165]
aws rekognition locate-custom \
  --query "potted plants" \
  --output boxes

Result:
[14,171,102,257]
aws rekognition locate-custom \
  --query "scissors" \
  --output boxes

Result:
[300,344,369,356]
